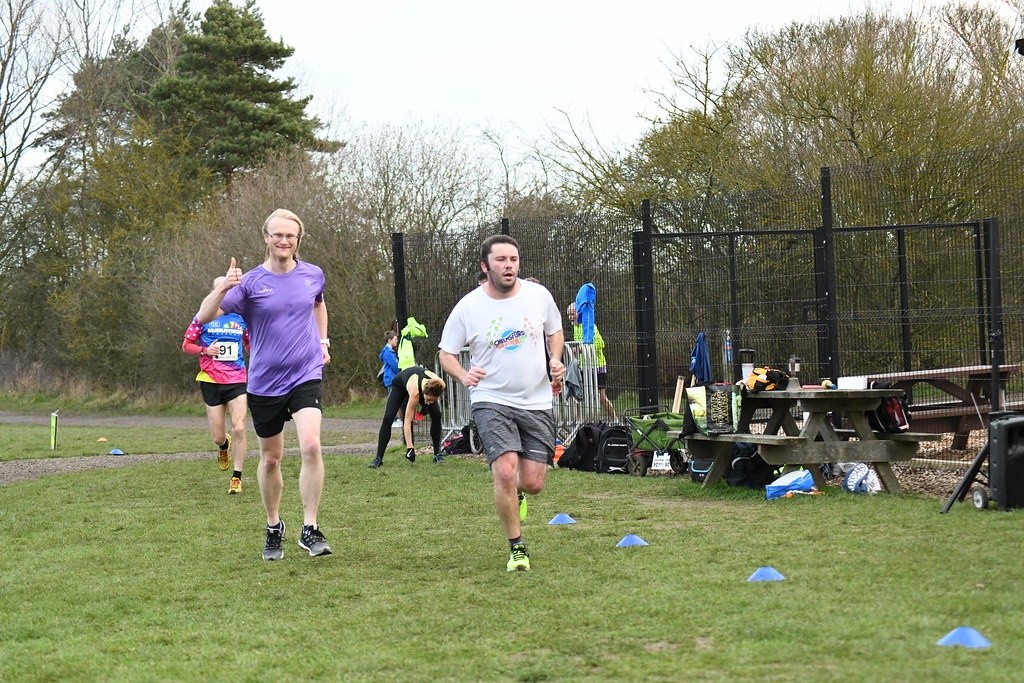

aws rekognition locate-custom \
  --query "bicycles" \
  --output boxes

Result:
[467,417,483,455]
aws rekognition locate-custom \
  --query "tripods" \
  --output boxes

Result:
[940,218,1009,515]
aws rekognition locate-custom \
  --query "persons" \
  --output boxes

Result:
[478,272,488,288]
[525,278,539,284]
[182,276,250,494]
[438,235,566,572]
[368,366,446,469]
[198,209,332,560]
[379,331,403,427]
[568,301,620,423]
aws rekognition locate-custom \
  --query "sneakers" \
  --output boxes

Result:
[218,434,231,470]
[517,489,527,523]
[261,517,287,561]
[433,453,445,465]
[506,544,532,573]
[368,457,383,468]
[228,477,242,494]
[298,524,332,557]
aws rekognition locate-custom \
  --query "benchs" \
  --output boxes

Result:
[664,398,1024,487]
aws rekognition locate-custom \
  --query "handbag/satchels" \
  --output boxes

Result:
[685,381,743,436]
[689,459,714,484]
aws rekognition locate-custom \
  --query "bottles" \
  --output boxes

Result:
[822,379,836,390]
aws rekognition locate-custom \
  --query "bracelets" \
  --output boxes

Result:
[320,339,331,348]
[201,348,205,356]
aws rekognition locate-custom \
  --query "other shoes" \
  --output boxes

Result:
[599,416,618,424]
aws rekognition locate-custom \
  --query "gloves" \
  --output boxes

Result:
[420,404,428,416]
[407,449,415,462]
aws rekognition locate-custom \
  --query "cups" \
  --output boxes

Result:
[742,363,754,384]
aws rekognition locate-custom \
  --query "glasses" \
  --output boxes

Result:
[265,231,299,241]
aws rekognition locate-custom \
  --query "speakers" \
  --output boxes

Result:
[989,414,1024,512]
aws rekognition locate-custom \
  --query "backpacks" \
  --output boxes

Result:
[864,380,909,433]
[576,422,604,472]
[723,443,772,491]
[594,428,632,475]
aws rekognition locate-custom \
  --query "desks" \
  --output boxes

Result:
[867,363,1022,449]
[726,386,906,493]
